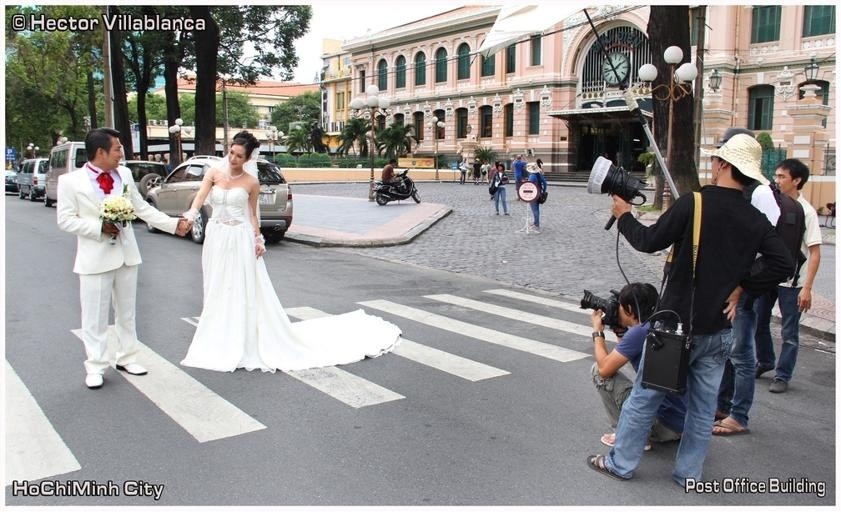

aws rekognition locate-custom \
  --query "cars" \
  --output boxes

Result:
[5,169,19,192]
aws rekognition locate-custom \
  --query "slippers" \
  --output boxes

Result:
[711,408,730,421]
[711,410,751,436]
[599,432,651,450]
[587,452,628,482]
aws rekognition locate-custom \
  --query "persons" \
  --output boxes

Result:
[754,157,823,394]
[472,157,481,185]
[458,158,470,186]
[56,127,194,388]
[586,132,797,491]
[712,126,806,436]
[381,157,404,185]
[511,155,528,199]
[588,281,688,452]
[486,158,501,201]
[480,158,491,184]
[177,129,403,375]
[492,163,511,216]
[526,158,547,230]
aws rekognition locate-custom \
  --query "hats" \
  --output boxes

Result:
[525,163,542,174]
[710,132,772,187]
[714,126,756,147]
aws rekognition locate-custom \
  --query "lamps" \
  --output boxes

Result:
[466,125,472,133]
[708,69,721,91]
[805,60,819,80]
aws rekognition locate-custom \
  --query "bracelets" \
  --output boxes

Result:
[591,330,606,342]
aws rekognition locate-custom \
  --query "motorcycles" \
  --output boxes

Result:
[373,171,422,205]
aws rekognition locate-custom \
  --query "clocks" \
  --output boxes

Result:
[602,52,631,85]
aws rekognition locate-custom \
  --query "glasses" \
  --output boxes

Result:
[770,174,792,180]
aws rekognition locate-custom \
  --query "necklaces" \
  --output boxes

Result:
[224,167,248,180]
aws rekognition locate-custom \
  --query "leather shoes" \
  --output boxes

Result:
[114,357,149,376]
[768,375,793,393]
[752,360,773,376]
[85,360,105,389]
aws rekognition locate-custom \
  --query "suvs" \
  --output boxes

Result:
[144,155,293,244]
[119,161,168,202]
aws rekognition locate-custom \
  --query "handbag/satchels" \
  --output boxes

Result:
[539,191,548,205]
[489,180,499,194]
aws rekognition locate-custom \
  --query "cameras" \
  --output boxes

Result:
[579,287,628,326]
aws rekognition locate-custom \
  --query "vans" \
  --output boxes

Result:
[18,159,47,201]
[45,141,89,206]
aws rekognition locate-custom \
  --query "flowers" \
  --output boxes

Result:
[100,196,136,243]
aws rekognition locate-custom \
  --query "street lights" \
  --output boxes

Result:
[167,119,191,166]
[348,84,390,204]
[425,114,445,181]
[639,44,697,218]
